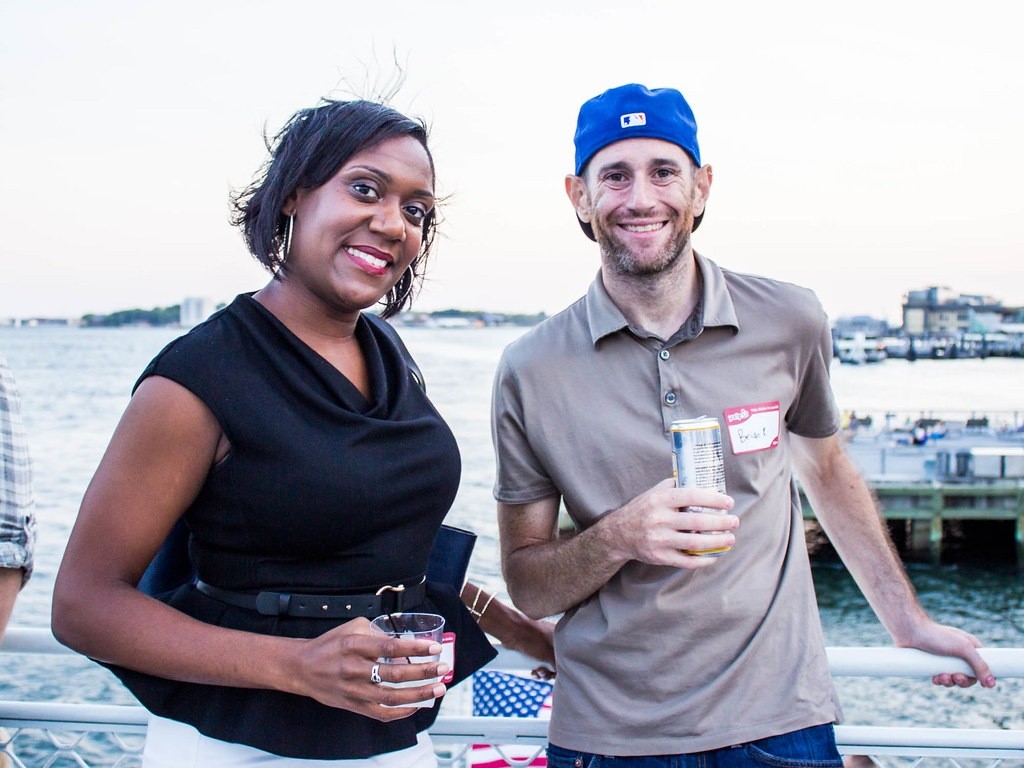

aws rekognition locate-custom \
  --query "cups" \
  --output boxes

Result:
[370,612,445,709]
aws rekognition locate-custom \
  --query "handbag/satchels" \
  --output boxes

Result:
[425,525,477,597]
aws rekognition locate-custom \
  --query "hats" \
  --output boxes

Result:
[573,83,706,242]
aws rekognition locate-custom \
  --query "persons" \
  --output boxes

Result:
[905,418,927,444]
[0,360,36,647]
[841,410,858,429]
[50,100,556,766]
[490,82,997,768]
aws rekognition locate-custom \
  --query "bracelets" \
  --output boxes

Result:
[460,578,496,624]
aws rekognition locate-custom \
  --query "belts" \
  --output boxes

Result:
[189,568,428,619]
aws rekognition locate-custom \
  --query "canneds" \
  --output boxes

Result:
[669,416,731,557]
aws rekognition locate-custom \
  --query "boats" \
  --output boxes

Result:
[836,332,888,366]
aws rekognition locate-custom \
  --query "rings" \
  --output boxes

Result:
[371,662,381,683]
